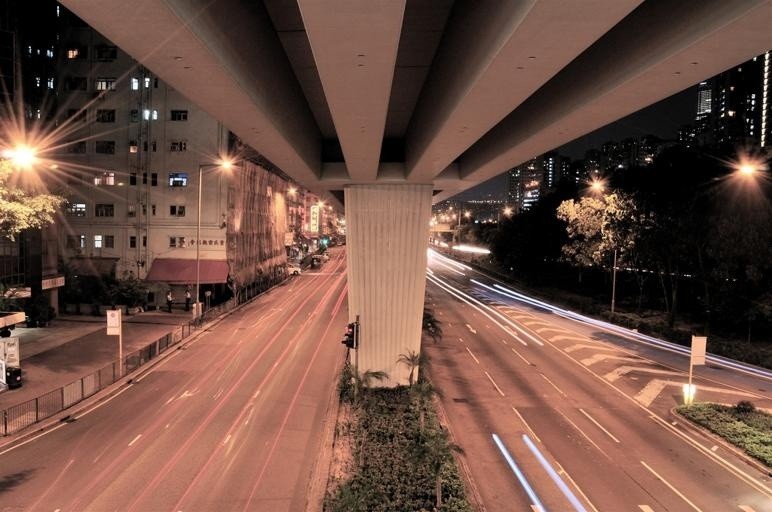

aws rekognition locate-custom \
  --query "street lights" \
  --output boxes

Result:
[195,157,231,320]
[591,179,618,314]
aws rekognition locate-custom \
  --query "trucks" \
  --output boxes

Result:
[311,252,330,269]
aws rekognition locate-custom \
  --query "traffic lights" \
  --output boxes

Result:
[341,322,354,348]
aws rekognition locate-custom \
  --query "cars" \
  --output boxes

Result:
[286,262,301,276]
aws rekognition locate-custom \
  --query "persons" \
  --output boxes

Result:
[184,290,191,311]
[166,291,175,313]
